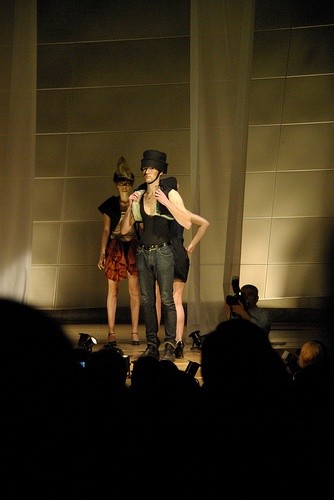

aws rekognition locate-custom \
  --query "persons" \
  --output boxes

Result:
[225,284,272,338]
[155,208,210,358]
[0,293,334,500]
[97,156,142,346]
[120,150,192,361]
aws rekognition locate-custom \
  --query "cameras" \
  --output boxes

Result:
[226,276,249,317]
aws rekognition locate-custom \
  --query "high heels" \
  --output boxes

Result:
[174,341,185,359]
[107,332,117,346]
[130,332,140,346]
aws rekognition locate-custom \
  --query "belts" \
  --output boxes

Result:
[138,241,172,251]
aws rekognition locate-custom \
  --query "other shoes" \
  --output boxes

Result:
[130,345,160,368]
[162,342,176,364]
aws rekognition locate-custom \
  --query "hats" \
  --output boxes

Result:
[111,156,135,186]
[140,149,168,175]
